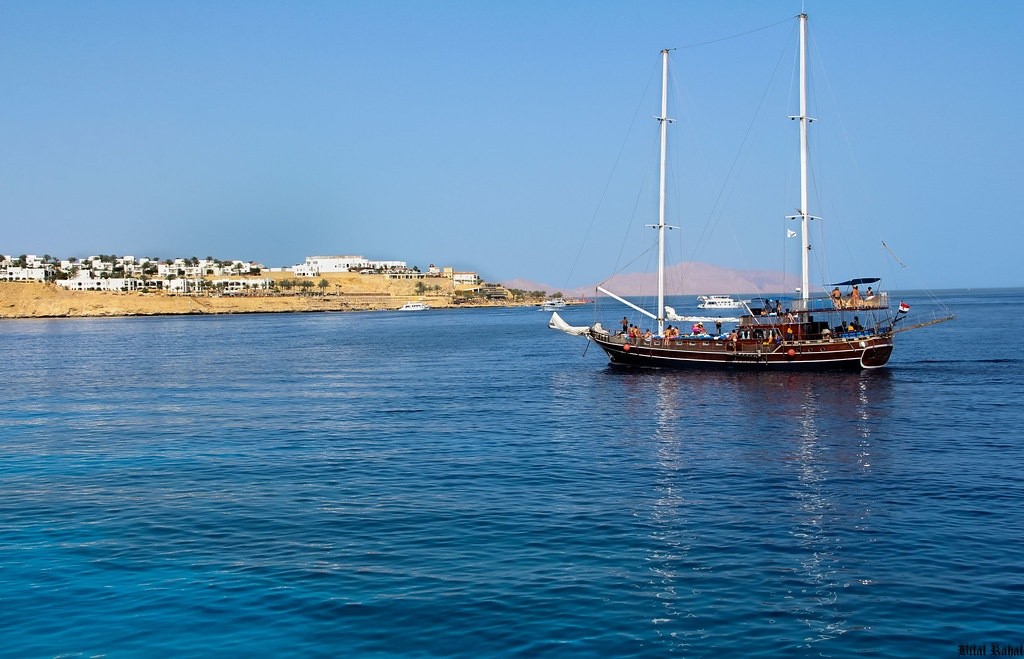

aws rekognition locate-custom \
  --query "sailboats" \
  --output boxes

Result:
[549,0,954,367]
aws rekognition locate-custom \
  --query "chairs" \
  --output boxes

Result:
[806,298,835,310]
[653,334,727,340]
[840,328,887,338]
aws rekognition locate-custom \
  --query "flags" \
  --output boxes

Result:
[899,303,910,314]
[787,230,796,237]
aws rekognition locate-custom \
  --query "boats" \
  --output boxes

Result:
[696,295,730,301]
[552,297,566,307]
[537,299,564,311]
[398,301,430,311]
[697,298,745,308]
[741,300,752,304]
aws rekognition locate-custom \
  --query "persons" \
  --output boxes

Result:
[664,325,679,347]
[730,325,792,352]
[866,287,873,298]
[837,321,863,332]
[714,316,722,335]
[851,286,859,299]
[691,322,706,335]
[620,317,653,338]
[831,287,841,300]
[761,299,796,323]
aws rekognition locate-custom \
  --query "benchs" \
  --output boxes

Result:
[841,300,879,308]
